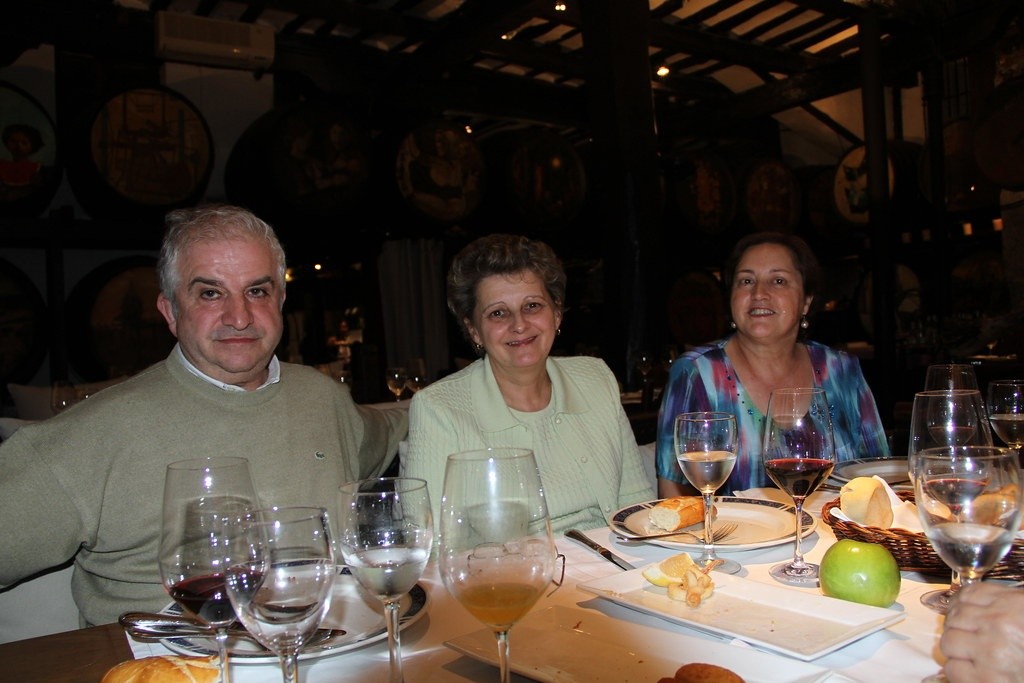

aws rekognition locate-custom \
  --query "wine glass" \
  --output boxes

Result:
[674,412,741,574]
[908,364,1024,613]
[337,477,433,683]
[158,455,270,683]
[439,447,555,683]
[763,387,835,588]
[225,506,336,683]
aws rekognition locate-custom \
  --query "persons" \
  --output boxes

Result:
[0,124,50,185]
[291,117,479,205]
[655,230,891,499]
[400,235,660,562]
[940,582,1024,683]
[0,204,410,632]
[326,319,352,360]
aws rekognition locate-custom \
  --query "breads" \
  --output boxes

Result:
[648,495,717,531]
[838,476,894,529]
[960,481,1024,532]
[99,653,223,683]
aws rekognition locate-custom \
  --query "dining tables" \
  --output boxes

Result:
[1,464,1023,682]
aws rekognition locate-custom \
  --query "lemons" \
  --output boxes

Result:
[641,553,700,587]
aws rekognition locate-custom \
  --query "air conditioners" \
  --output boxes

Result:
[155,11,275,68]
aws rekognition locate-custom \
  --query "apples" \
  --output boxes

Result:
[819,539,901,609]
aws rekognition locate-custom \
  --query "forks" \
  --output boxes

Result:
[617,522,738,542]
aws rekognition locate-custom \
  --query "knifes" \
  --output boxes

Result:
[564,529,637,571]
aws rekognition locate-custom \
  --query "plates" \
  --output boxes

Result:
[158,565,429,663]
[443,606,832,683]
[830,456,914,489]
[581,563,908,662]
[609,496,817,553]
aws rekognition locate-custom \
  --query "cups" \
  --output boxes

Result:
[48,377,77,413]
[386,358,426,401]
